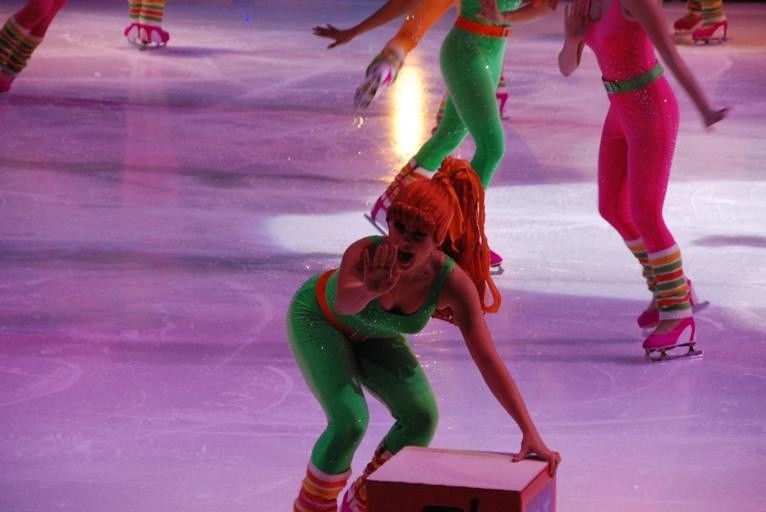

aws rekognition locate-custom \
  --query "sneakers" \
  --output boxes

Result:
[353,61,394,112]
[673,11,728,38]
[637,277,696,350]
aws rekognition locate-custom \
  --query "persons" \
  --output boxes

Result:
[123,0,170,49]
[558,0,730,350]
[0,0,70,92]
[286,155,561,512]
[312,0,560,267]
[674,0,729,41]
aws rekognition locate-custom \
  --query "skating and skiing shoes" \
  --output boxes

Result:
[124,21,169,43]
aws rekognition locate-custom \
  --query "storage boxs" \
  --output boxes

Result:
[366,444,558,512]
